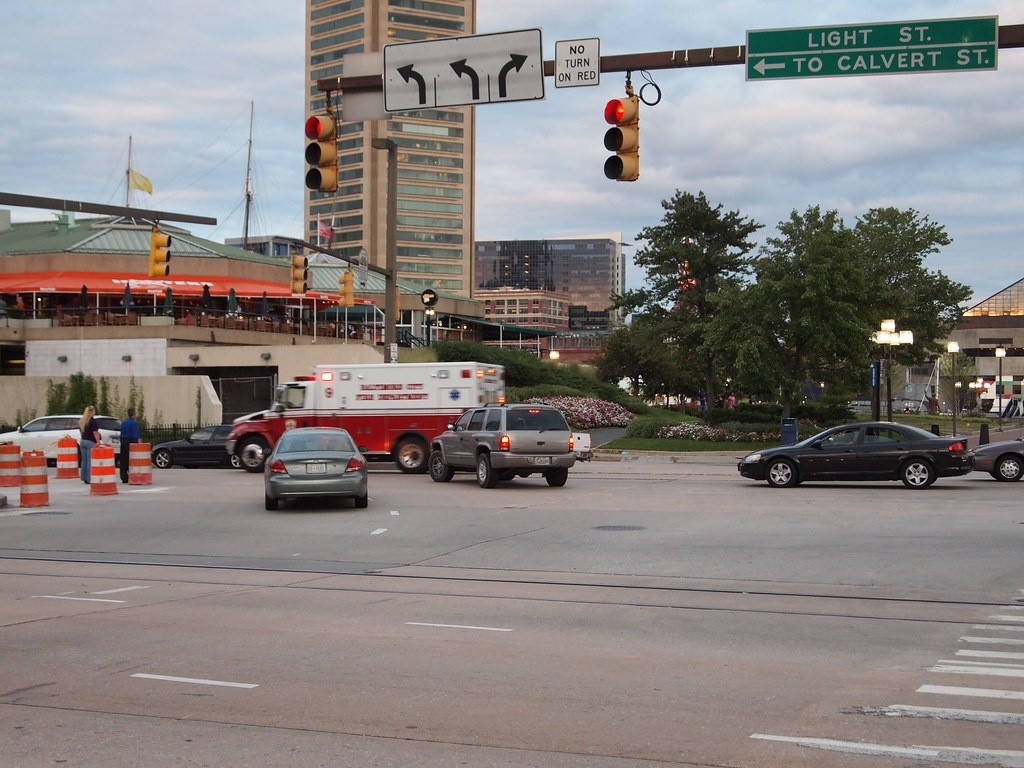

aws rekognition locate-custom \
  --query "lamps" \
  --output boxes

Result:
[260,351,271,360]
[57,356,68,362]
[188,354,199,361]
[121,355,132,362]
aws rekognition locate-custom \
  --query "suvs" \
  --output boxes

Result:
[429,401,577,489]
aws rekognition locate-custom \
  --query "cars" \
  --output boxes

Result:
[150,425,243,469]
[968,435,1024,482]
[0,414,122,467]
[737,421,975,489]
[264,426,369,511]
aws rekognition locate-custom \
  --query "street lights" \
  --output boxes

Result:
[867,320,913,438]
[954,381,961,416]
[968,377,990,418]
[947,342,959,438]
[421,288,438,348]
[996,348,1006,432]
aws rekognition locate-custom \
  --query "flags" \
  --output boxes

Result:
[319,219,332,240]
[128,169,152,194]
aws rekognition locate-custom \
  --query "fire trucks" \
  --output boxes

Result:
[225,362,507,473]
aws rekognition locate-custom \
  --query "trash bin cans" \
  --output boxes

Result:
[979,424,989,444]
[931,425,940,436]
[780,418,798,445]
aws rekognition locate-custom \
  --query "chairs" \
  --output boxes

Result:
[326,438,346,451]
[867,432,876,442]
[288,440,308,451]
[59,312,381,341]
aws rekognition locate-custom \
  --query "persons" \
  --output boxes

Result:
[923,389,941,415]
[77,406,100,483]
[120,408,141,482]
[697,387,755,415]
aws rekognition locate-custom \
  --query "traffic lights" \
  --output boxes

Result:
[305,113,338,193]
[604,98,641,182]
[148,232,171,277]
[338,273,355,308]
[291,254,308,294]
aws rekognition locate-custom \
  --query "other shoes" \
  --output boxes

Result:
[84,480,90,484]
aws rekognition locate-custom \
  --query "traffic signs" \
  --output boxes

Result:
[745,13,999,81]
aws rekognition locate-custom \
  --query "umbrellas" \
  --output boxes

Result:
[227,288,238,314]
[120,284,134,308]
[81,284,88,310]
[260,291,269,318]
[203,285,211,312]
[162,287,174,316]
